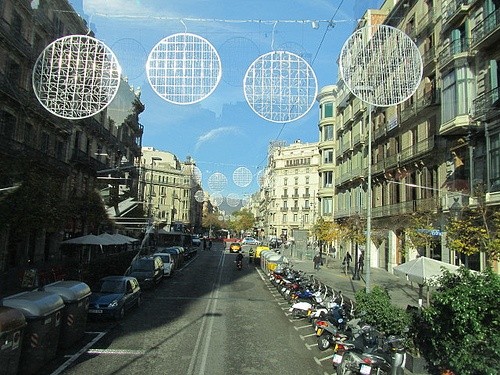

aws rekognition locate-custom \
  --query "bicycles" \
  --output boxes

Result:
[314,262,321,271]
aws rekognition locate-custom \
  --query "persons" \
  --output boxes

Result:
[208,239,212,250]
[359,254,364,273]
[331,246,336,258]
[235,251,243,268]
[202,238,206,250]
[344,252,352,268]
[313,254,322,272]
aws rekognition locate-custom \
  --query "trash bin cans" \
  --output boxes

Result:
[261,251,283,274]
[0,307,26,375]
[46,280,92,348]
[3,289,65,370]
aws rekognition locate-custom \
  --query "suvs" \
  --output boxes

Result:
[130,256,164,290]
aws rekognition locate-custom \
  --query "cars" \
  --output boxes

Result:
[241,232,262,245]
[229,243,241,253]
[153,246,186,276]
[90,276,145,320]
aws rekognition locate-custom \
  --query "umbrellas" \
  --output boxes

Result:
[111,234,140,256]
[392,256,486,309]
[97,232,133,259]
[59,232,116,262]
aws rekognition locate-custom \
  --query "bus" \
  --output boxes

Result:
[216,228,229,238]
[190,239,202,249]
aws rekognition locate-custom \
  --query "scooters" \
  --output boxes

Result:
[270,265,329,318]
[313,302,409,375]
[236,259,243,271]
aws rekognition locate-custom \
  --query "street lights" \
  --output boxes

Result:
[352,211,360,281]
[450,197,462,287]
